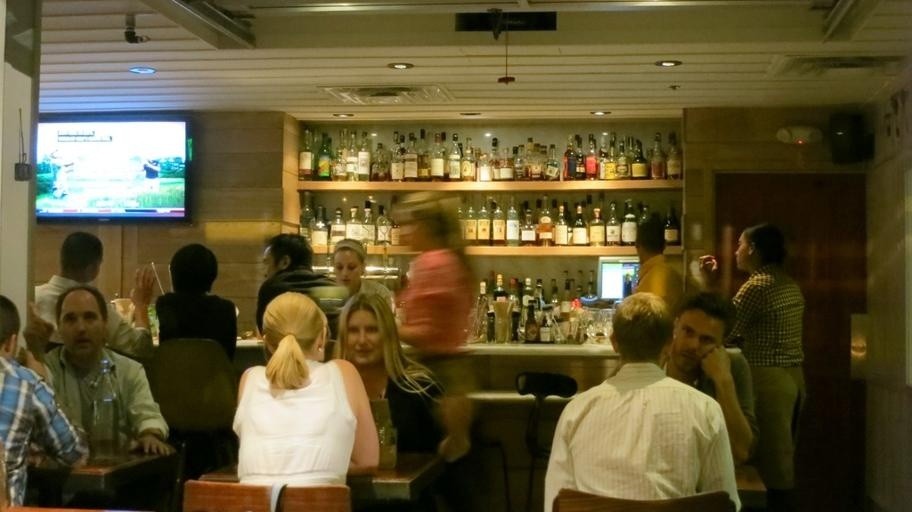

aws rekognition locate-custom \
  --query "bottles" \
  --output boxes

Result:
[91,359,121,469]
[300,126,370,181]
[457,136,562,181]
[391,127,461,179]
[479,270,572,344]
[522,192,678,246]
[563,131,682,181]
[301,190,401,245]
[370,142,389,178]
[459,192,522,247]
[575,269,597,300]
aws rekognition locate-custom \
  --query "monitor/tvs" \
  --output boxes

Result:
[596,255,641,307]
[33,112,194,224]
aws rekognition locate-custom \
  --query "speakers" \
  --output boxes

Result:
[828,110,874,166]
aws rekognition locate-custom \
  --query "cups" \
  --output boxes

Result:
[376,424,398,470]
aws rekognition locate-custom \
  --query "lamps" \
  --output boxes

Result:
[141,0,254,49]
[822,0,883,43]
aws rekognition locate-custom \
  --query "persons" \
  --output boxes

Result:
[256,231,352,337]
[334,291,471,469]
[544,292,742,512]
[42,287,171,512]
[156,243,238,481]
[333,238,397,309]
[394,209,475,397]
[0,297,89,510]
[635,222,684,307]
[28,231,153,357]
[232,292,380,486]
[698,224,805,490]
[666,292,756,461]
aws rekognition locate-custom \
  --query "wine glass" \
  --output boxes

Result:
[584,307,602,345]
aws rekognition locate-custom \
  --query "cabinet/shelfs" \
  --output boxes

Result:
[296,120,686,401]
